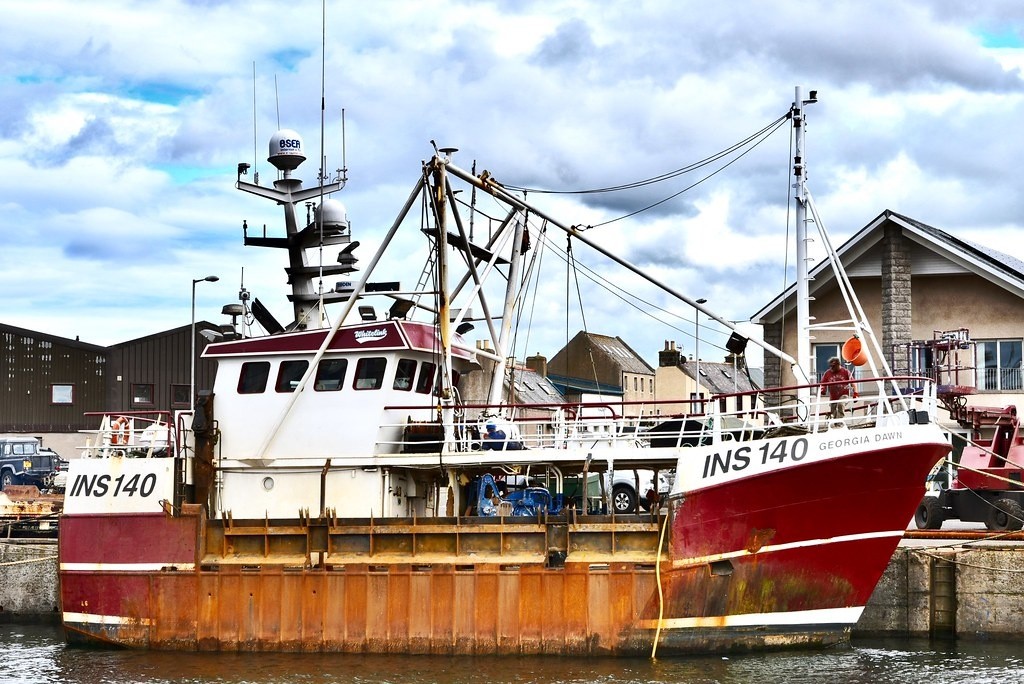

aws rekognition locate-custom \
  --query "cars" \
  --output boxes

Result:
[0,437,59,490]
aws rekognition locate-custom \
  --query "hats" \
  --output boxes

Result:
[486,425,496,429]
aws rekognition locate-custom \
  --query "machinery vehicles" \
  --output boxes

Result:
[913,327,1024,533]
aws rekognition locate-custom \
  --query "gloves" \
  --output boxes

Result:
[853,392,859,403]
[821,388,826,395]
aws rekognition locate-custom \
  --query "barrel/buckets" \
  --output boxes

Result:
[842,336,868,366]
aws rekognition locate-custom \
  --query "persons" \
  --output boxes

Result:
[481,421,530,451]
[820,357,859,428]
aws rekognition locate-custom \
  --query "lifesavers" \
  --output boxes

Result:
[111,415,129,446]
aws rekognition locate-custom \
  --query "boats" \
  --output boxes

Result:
[59,0,955,655]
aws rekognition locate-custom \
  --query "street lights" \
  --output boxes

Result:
[694,298,708,412]
[191,276,220,410]
